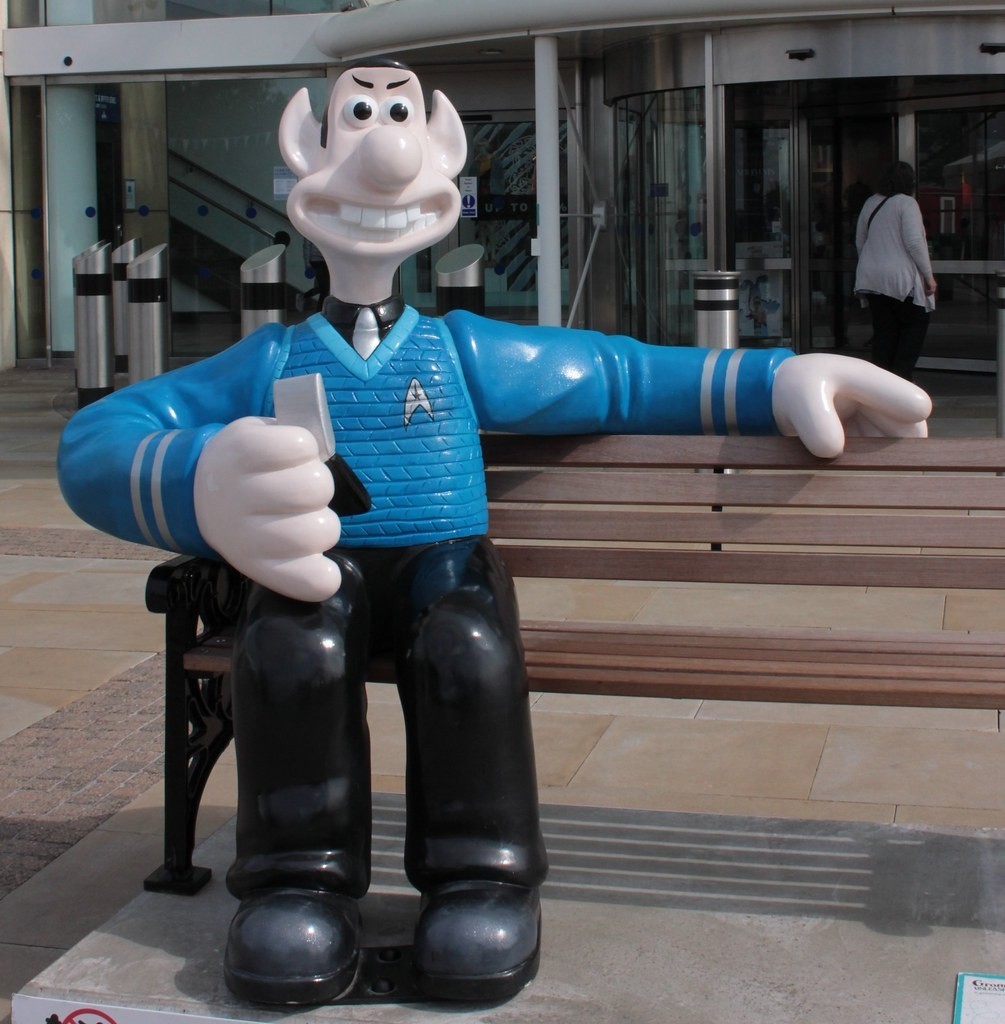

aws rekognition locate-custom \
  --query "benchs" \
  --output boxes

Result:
[145,432,1005,899]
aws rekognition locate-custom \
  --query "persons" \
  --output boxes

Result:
[57,59,933,1009]
[854,163,938,387]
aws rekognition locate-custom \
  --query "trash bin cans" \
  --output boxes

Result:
[689,270,742,474]
